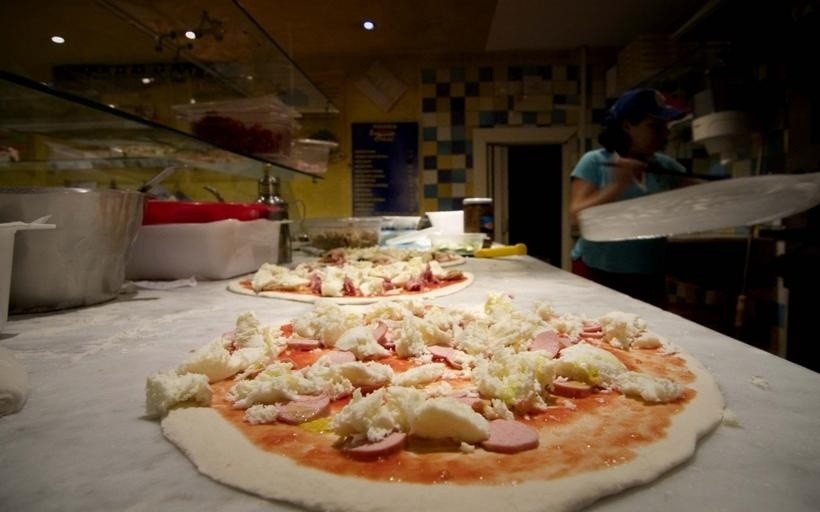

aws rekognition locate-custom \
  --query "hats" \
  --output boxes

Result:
[605,88,692,120]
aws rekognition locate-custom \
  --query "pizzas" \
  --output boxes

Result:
[145,295,723,512]
[228,264,474,305]
[319,247,465,267]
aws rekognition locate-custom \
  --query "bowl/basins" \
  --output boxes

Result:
[144,199,283,223]
[430,231,488,257]
[2,184,147,312]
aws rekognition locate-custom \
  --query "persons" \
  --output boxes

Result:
[564,89,703,302]
